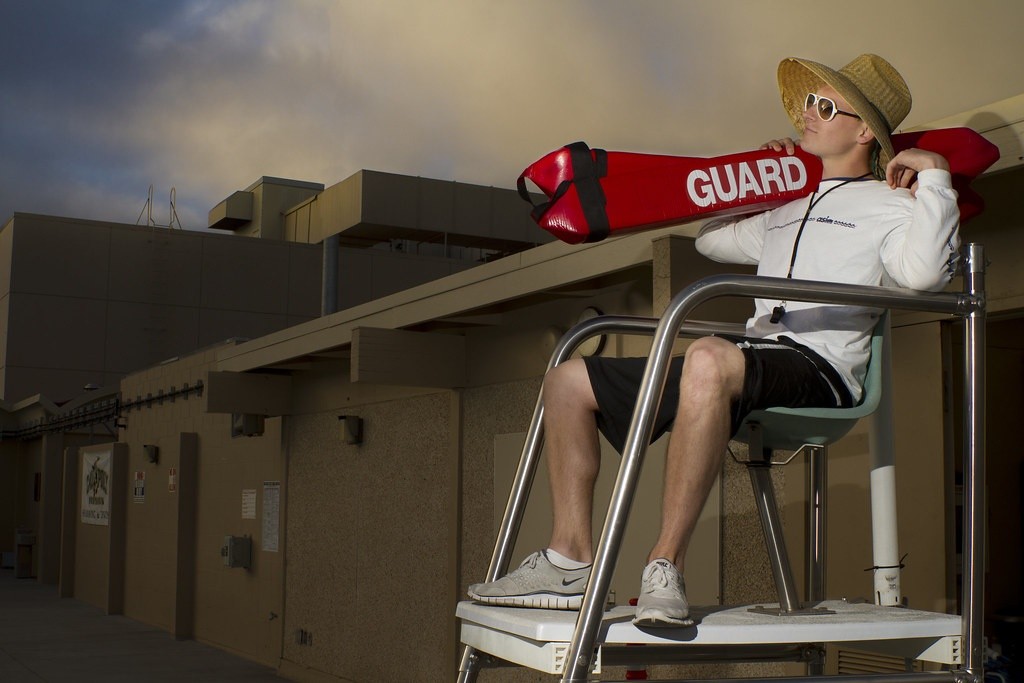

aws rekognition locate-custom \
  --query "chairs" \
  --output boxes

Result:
[730,305,889,618]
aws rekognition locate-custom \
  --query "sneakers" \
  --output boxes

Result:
[632,557,695,627]
[466,549,594,610]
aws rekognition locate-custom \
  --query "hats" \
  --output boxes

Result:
[777,51,912,182]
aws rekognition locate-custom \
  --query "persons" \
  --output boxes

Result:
[466,51,963,631]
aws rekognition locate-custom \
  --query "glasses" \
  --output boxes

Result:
[804,91,863,122]
